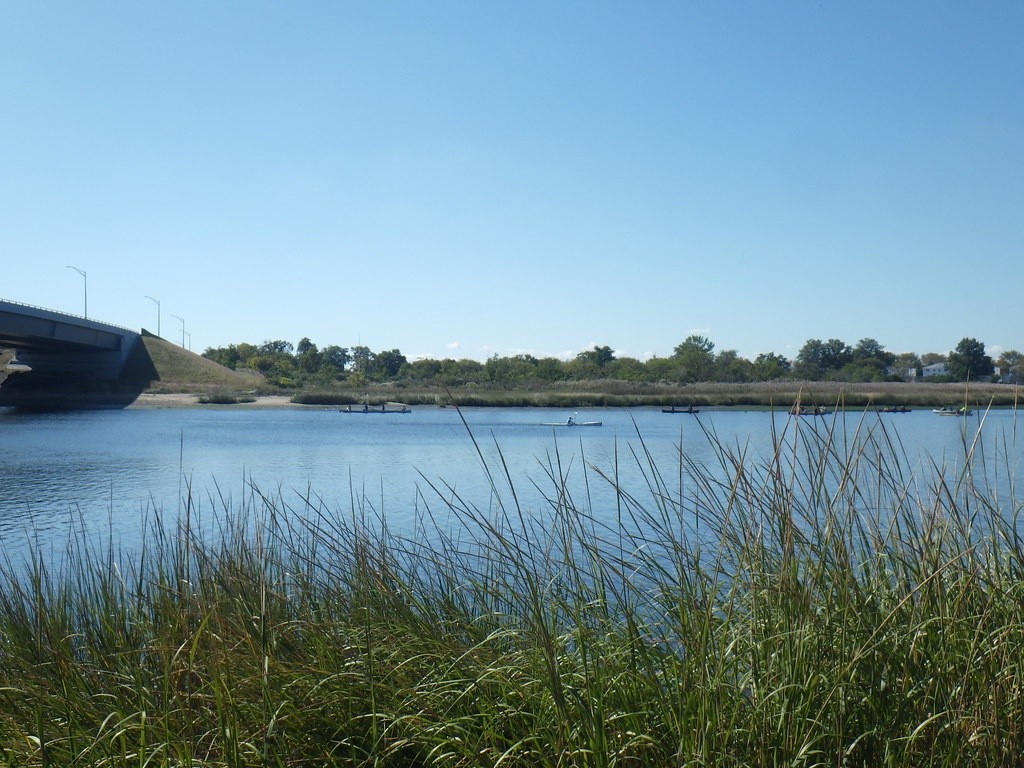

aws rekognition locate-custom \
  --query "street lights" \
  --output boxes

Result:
[179,329,190,351]
[66,266,87,319]
[172,315,184,349]
[144,296,161,337]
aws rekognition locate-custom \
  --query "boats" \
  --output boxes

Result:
[540,421,602,426]
[661,408,700,413]
[339,409,412,413]
[933,408,956,413]
[788,410,832,415]
[940,412,973,416]
[877,408,912,412]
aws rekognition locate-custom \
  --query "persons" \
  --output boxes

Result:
[364,404,369,410]
[382,404,385,410]
[819,404,826,412]
[348,404,351,411]
[566,416,574,424]
